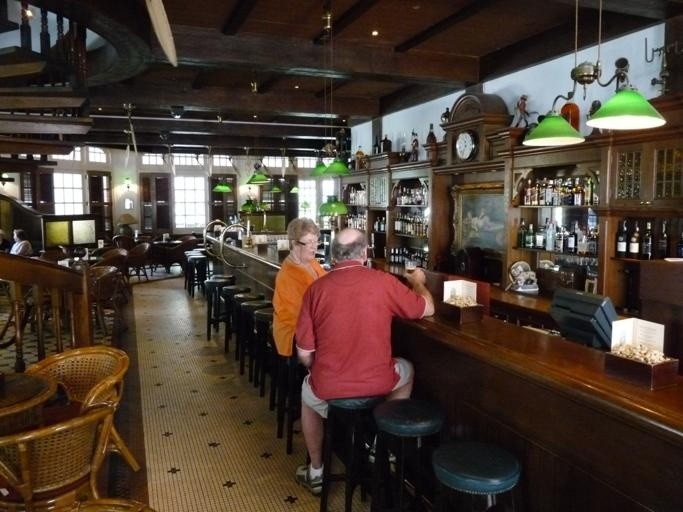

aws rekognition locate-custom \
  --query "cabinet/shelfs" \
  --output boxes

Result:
[507,128,683,309]
[333,152,452,275]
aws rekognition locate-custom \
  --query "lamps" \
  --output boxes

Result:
[270,184,281,193]
[317,201,348,231]
[211,181,233,194]
[308,159,326,178]
[246,171,270,185]
[323,158,351,175]
[520,0,668,148]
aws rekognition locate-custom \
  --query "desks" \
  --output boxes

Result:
[0,366,58,425]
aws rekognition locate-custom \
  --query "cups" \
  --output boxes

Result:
[97,240,103,249]
[161,232,169,243]
[405,261,415,273]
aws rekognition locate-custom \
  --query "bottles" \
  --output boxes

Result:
[347,122,436,171]
[396,184,424,206]
[389,246,425,267]
[523,170,599,206]
[515,215,598,258]
[346,188,368,204]
[614,220,681,262]
[560,92,579,132]
[373,217,384,232]
[344,213,367,231]
[393,213,427,237]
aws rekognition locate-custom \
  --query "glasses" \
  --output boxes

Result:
[296,239,323,249]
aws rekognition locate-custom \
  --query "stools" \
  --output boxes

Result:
[203,272,311,454]
[318,396,385,510]
[183,247,208,297]
[430,437,522,512]
[369,398,447,510]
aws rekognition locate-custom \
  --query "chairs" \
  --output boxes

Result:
[25,345,142,474]
[0,405,117,511]
[112,232,200,283]
[1,244,129,330]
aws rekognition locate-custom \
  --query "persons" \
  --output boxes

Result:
[269,217,329,357]
[9,228,33,257]
[295,227,437,496]
[0,229,10,255]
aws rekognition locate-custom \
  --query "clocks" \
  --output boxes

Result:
[454,130,478,163]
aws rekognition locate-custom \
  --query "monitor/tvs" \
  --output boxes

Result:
[550,287,619,352]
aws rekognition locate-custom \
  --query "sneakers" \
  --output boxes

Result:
[294,463,324,496]
[368,444,397,464]
